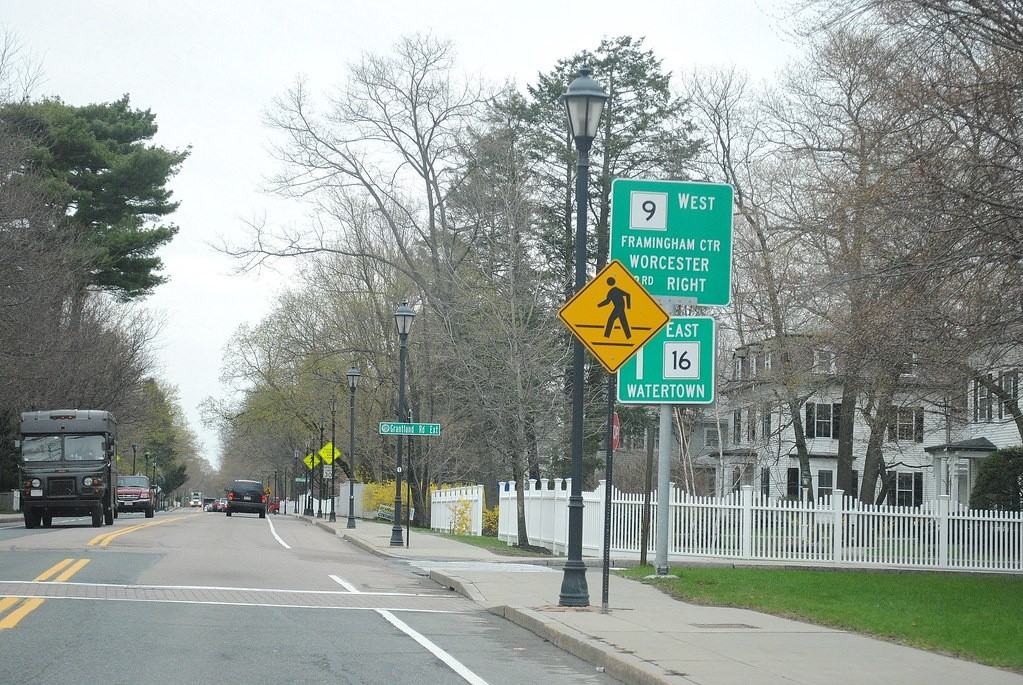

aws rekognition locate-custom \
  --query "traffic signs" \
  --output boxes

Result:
[617,317,716,405]
[609,175,733,308]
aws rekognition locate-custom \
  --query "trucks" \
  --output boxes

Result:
[268,498,279,515]
[11,410,118,529]
[190,492,202,507]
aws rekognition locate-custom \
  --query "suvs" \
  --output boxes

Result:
[114,476,155,518]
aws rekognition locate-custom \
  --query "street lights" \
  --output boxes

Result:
[556,63,611,607]
[145,454,150,476]
[153,461,157,476]
[389,304,417,548]
[345,366,362,529]
[293,414,325,518]
[132,445,138,475]
[327,395,339,523]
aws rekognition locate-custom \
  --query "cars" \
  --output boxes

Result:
[224,480,270,518]
[203,497,227,512]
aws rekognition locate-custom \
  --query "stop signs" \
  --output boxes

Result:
[613,413,620,453]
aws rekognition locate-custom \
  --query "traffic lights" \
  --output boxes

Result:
[186,496,189,498]
